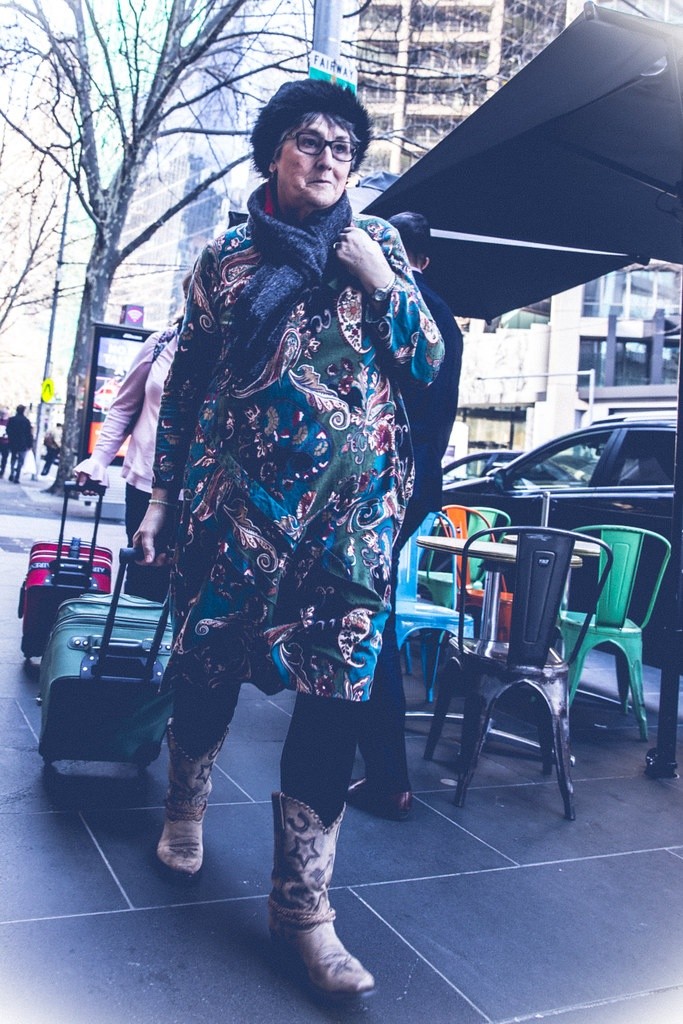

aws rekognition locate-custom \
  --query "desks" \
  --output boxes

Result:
[404,536,582,767]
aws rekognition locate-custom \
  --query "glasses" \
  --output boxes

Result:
[285,130,360,162]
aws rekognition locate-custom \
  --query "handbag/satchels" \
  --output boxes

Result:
[22,447,37,475]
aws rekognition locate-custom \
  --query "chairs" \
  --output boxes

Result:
[396,504,671,820]
[504,535,600,557]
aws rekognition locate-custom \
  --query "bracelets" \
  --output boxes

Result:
[148,499,180,510]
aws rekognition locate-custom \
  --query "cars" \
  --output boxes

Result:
[417,417,682,651]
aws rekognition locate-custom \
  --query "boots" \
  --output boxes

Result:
[267,791,378,1004]
[151,723,230,885]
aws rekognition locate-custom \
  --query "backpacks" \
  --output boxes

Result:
[43,431,53,447]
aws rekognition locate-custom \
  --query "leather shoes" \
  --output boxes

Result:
[344,777,413,822]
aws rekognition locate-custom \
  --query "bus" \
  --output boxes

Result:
[88,376,132,457]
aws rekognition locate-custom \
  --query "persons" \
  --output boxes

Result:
[130,82,444,1002]
[41,421,63,475]
[75,278,187,605]
[343,213,465,822]
[5,404,35,484]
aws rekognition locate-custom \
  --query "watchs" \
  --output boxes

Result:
[367,272,397,303]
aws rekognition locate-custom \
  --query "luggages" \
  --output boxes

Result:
[15,477,113,659]
[36,544,179,778]
